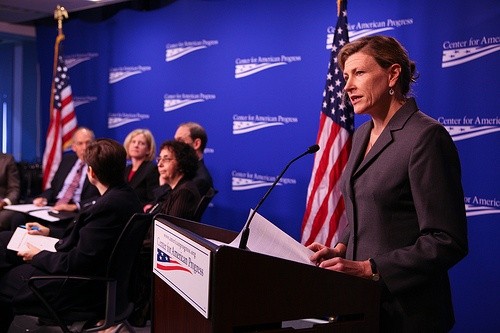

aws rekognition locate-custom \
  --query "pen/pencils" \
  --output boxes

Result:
[20,225,40,232]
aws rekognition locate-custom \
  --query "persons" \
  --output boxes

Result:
[0,121,214,309]
[307,35,468,333]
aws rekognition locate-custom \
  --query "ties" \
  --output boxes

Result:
[55,160,85,205]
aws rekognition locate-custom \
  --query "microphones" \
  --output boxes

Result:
[239,144,320,251]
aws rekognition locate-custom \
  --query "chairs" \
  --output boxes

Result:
[17,162,219,333]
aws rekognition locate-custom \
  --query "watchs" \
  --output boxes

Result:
[369,257,380,282]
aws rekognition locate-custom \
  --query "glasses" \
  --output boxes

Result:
[155,156,174,163]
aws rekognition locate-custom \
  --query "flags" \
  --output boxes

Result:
[300,0,354,247]
[41,55,79,192]
